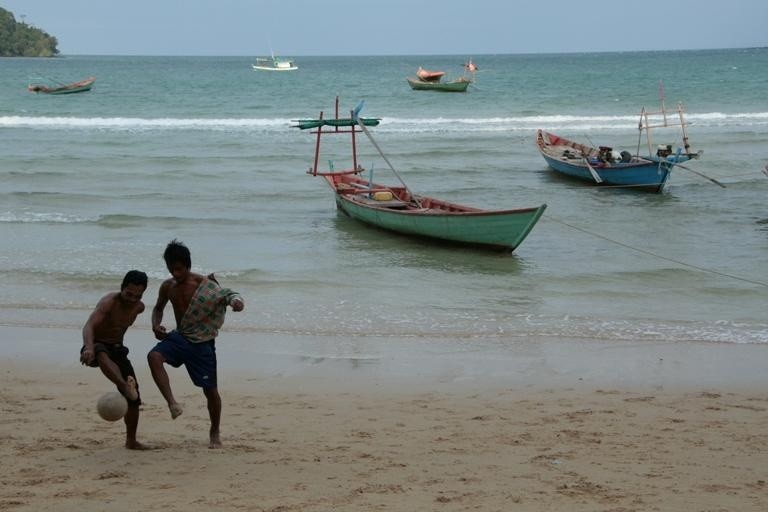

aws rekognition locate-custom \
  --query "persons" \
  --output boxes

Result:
[77,269,154,452]
[143,240,244,449]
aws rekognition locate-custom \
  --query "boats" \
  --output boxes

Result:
[249,51,299,72]
[404,63,472,94]
[462,60,479,72]
[23,75,97,94]
[530,86,706,195]
[289,89,549,256]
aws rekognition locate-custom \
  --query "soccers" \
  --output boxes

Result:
[98,392,128,422]
